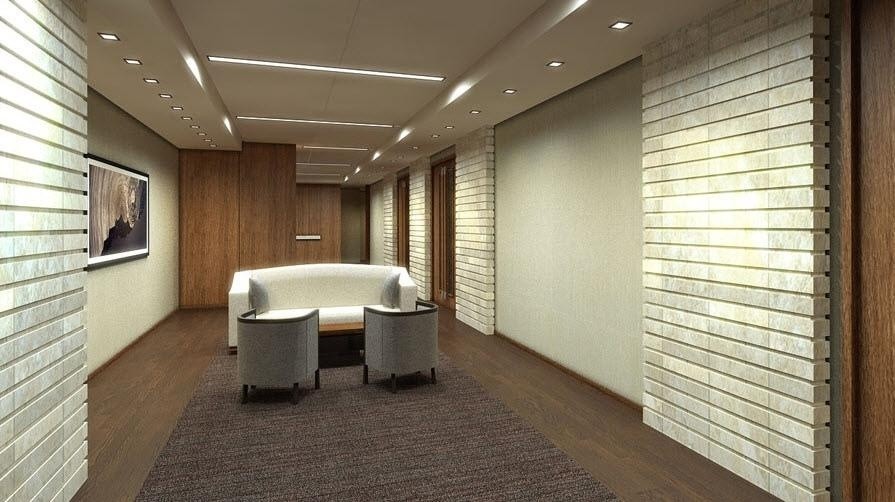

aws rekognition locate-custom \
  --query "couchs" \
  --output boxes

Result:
[227,261,418,355]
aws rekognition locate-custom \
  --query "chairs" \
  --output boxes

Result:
[359,300,439,393]
[236,309,320,405]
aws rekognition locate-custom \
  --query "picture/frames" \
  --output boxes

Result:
[84,152,149,270]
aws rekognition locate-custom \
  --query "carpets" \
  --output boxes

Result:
[135,332,617,502]
